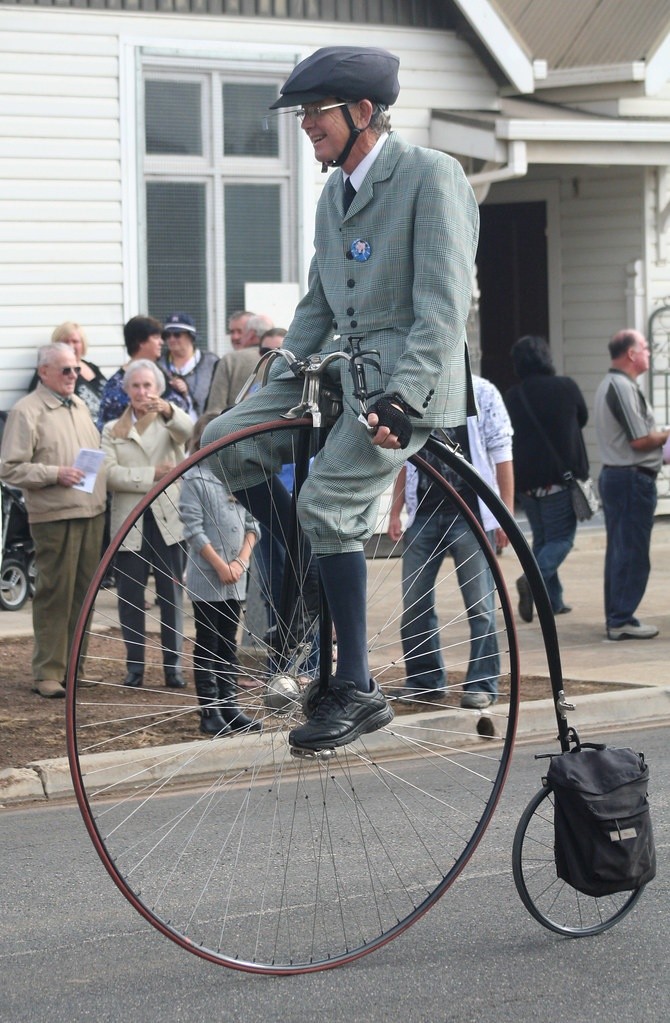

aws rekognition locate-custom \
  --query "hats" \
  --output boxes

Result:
[268,47,400,108]
[163,315,197,339]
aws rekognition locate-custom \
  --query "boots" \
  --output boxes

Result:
[217,658,263,729]
[194,659,233,734]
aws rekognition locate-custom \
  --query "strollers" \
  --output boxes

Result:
[0,481,39,610]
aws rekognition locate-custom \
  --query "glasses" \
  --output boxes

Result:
[45,363,79,378]
[295,96,358,125]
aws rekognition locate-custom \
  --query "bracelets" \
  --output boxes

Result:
[234,557,248,571]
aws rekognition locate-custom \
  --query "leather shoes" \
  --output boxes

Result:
[126,673,142,690]
[167,672,184,686]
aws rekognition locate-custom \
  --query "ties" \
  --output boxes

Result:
[344,177,354,213]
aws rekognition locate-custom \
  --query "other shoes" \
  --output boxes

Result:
[553,603,572,613]
[517,575,534,624]
[76,675,103,687]
[460,688,495,710]
[388,683,446,703]
[32,677,65,699]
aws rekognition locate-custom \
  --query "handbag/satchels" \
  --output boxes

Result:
[547,748,656,896]
[566,470,600,521]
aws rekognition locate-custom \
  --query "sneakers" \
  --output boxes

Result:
[287,675,395,749]
[265,579,324,648]
[606,620,659,641]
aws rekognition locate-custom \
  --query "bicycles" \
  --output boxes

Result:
[60,320,656,981]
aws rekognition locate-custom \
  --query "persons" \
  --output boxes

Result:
[0,342,109,698]
[179,412,264,735]
[387,375,514,709]
[100,309,338,686]
[502,335,590,622]
[593,329,670,641]
[28,321,108,426]
[201,44,480,748]
